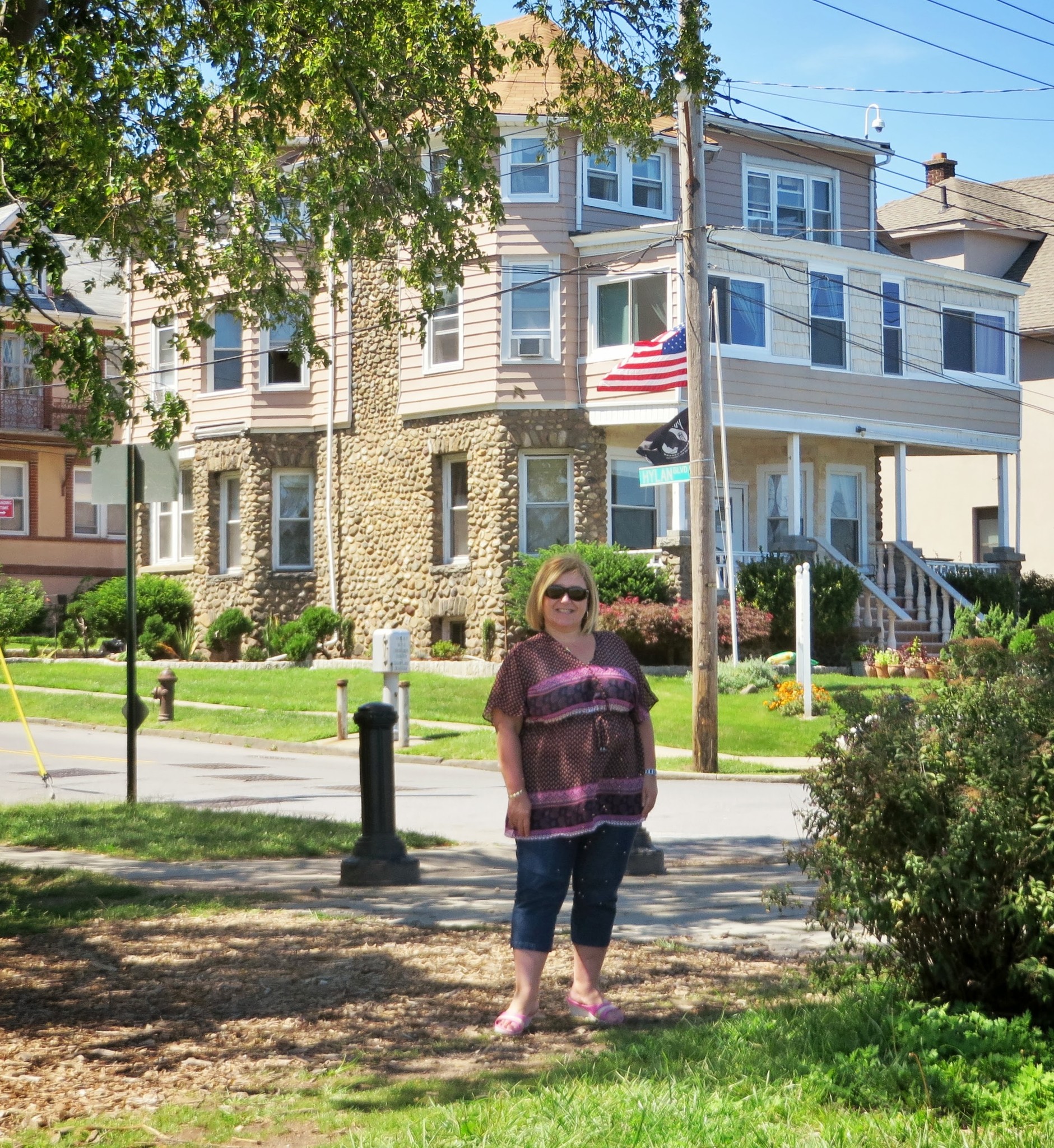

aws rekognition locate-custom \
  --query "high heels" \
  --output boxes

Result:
[494,1009,533,1035]
[567,995,624,1024]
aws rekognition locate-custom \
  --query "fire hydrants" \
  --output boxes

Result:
[150,665,178,721]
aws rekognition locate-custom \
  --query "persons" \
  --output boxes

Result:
[483,554,659,1036]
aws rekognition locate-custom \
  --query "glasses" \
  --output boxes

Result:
[544,583,589,601]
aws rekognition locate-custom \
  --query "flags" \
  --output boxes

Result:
[597,323,687,392]
[636,408,690,465]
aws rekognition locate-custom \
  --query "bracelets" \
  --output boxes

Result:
[508,787,526,799]
[641,768,656,776]
